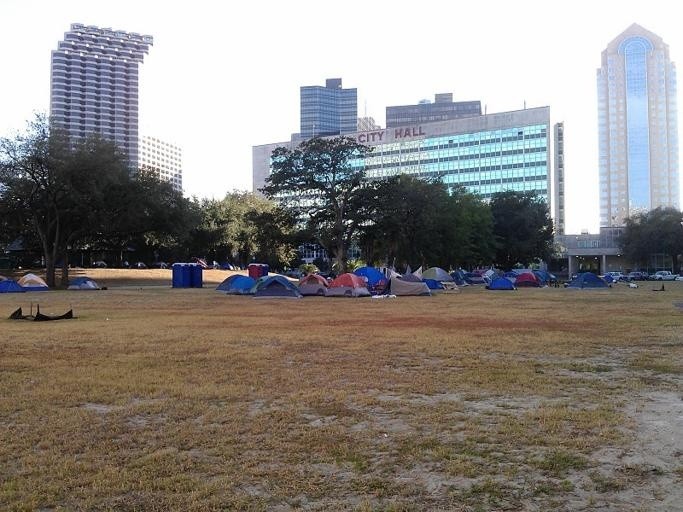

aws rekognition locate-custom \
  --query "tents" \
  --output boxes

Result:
[65,277,100,290]
[215,262,233,269]
[450,268,557,290]
[567,271,616,288]
[213,266,460,297]
[0,279,26,293]
[16,273,49,291]
[207,261,220,269]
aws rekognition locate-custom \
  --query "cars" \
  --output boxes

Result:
[604,270,682,281]
[0,253,269,270]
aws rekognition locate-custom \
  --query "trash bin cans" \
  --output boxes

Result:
[247,263,269,280]
[172,263,203,288]
